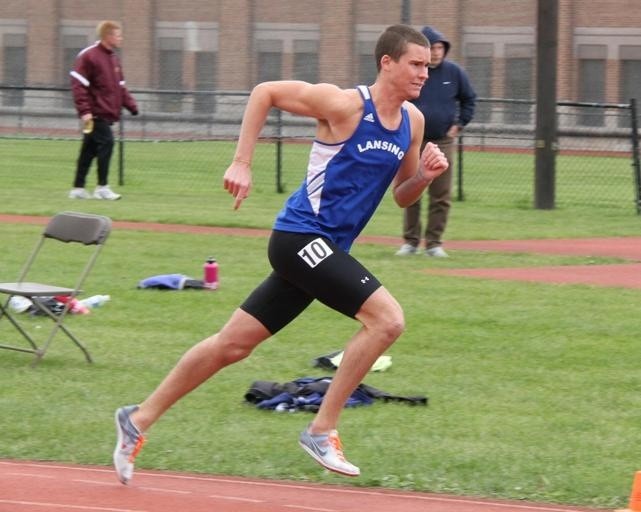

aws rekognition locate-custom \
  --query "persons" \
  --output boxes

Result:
[114,24,449,485]
[394,27,476,257]
[68,19,139,200]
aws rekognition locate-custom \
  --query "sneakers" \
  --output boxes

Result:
[113,405,145,486]
[69,187,91,199]
[93,185,121,200]
[298,429,361,478]
[395,243,419,256]
[425,247,447,257]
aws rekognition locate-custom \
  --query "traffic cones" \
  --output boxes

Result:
[625,469,641,512]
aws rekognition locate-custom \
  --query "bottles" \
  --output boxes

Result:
[201,256,219,291]
[79,291,109,306]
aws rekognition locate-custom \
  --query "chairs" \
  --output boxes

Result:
[3,210,116,368]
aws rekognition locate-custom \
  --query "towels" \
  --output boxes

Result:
[137,273,196,290]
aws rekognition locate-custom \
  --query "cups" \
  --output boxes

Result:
[79,119,95,135]
